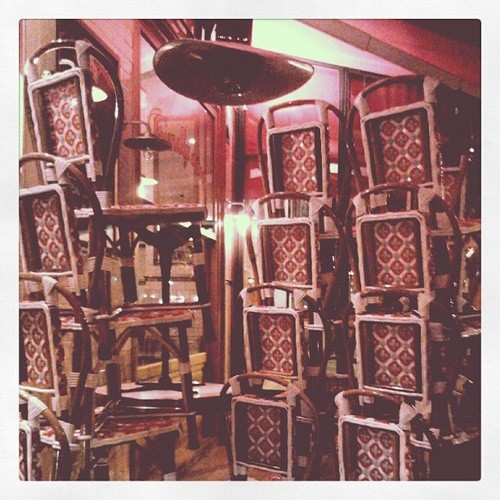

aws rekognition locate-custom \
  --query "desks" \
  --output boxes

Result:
[96,383,234,480]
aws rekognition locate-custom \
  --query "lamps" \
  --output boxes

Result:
[123,121,171,160]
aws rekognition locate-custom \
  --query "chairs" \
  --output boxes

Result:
[19,41,481,480]
